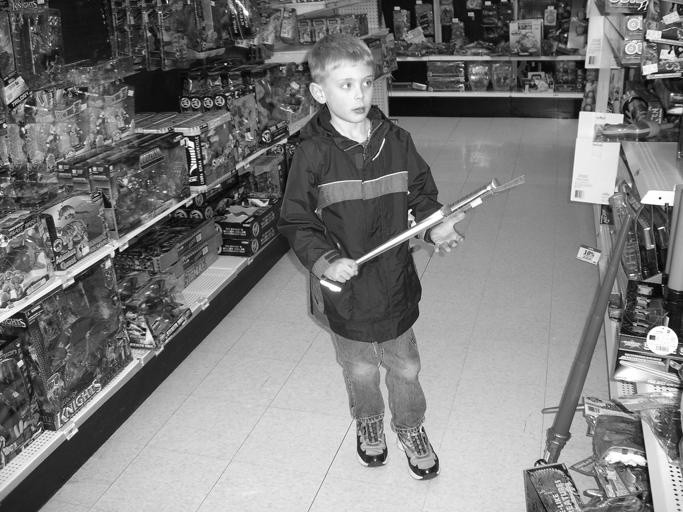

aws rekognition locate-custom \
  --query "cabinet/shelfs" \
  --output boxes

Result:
[0,0,388,512]
[388,1,586,119]
[637,191,682,512]
[594,1,683,236]
[596,142,680,399]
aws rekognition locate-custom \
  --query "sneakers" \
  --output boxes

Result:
[355,419,388,467]
[398,428,440,480]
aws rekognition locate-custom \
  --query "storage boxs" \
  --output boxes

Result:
[523,463,583,511]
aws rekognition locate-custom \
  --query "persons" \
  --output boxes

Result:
[277,33,467,481]
